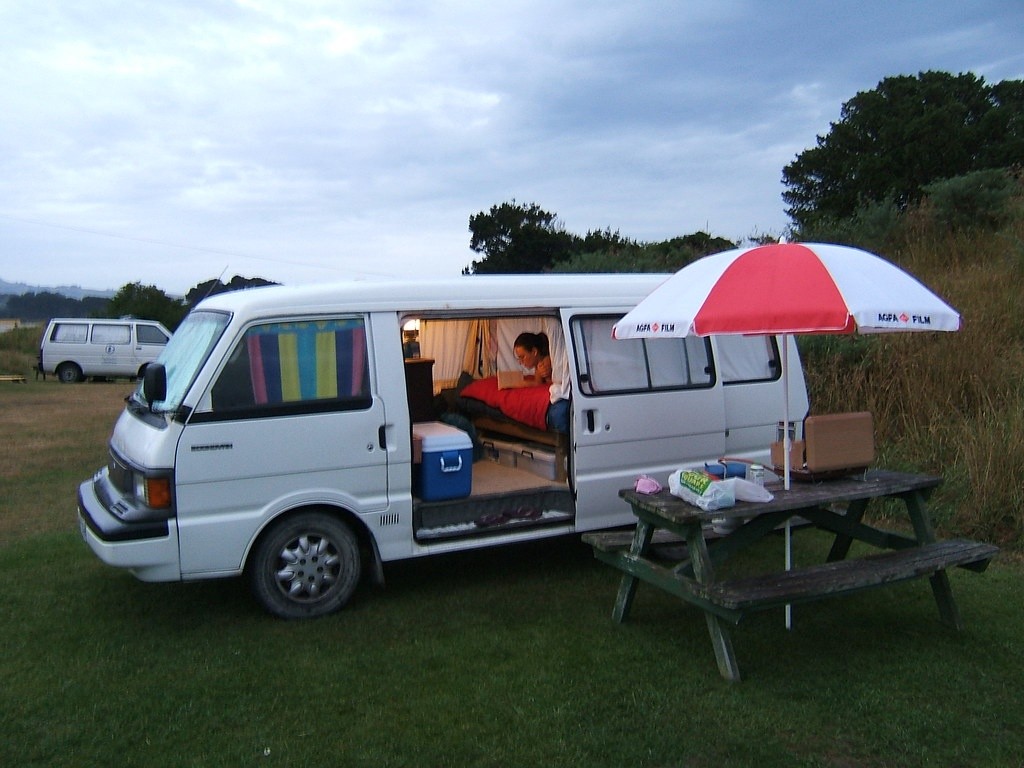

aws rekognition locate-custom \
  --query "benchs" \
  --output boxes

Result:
[580,509,848,553]
[686,536,1000,609]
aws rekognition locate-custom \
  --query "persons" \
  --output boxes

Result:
[403,335,413,358]
[514,331,552,385]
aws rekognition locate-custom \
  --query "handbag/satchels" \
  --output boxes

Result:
[668,468,774,512]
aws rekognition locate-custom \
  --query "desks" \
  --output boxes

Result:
[612,465,963,681]
[405,357,435,421]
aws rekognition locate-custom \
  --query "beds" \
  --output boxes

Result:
[436,372,570,484]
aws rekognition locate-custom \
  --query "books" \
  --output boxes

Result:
[497,371,529,391]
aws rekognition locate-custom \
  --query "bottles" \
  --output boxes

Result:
[749,461,764,490]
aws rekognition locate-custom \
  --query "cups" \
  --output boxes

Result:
[776,420,795,444]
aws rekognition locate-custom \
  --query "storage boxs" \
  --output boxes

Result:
[771,410,876,473]
[512,443,568,481]
[478,433,528,467]
[413,421,474,501]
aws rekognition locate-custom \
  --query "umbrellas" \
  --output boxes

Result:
[608,234,960,634]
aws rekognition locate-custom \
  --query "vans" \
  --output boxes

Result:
[38,318,172,384]
[76,274,811,620]
